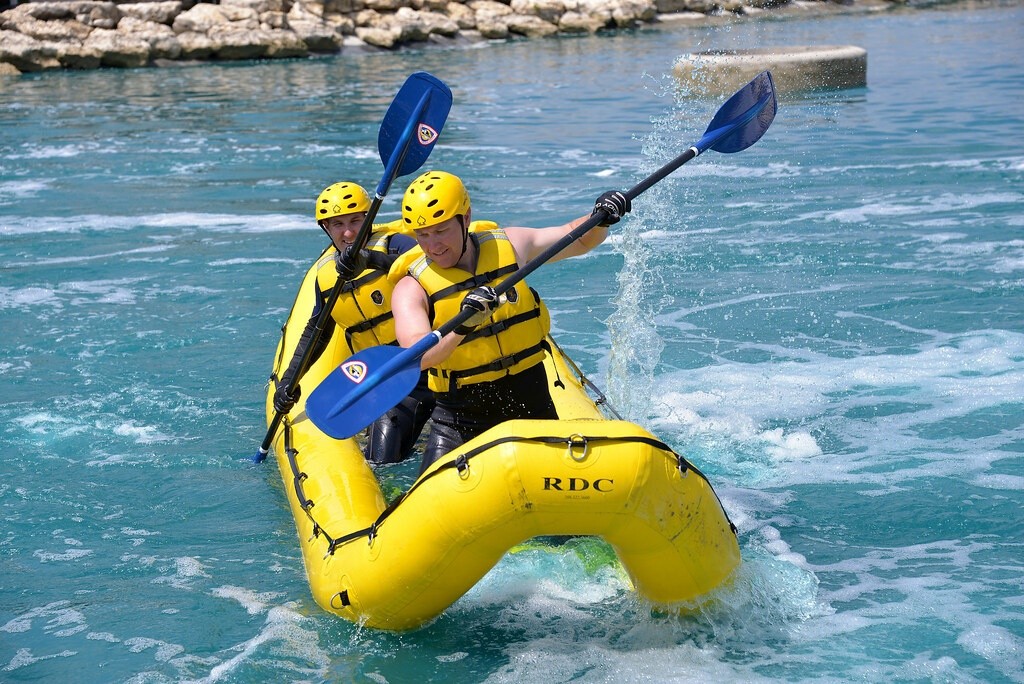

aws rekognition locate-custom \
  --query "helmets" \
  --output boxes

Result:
[401,171,470,230]
[315,182,371,222]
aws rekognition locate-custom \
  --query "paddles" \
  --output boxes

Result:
[253,74,454,465]
[303,68,778,442]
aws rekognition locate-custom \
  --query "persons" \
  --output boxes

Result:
[390,172,632,545]
[273,182,437,466]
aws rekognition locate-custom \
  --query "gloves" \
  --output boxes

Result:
[274,378,301,414]
[335,244,370,281]
[591,191,631,227]
[452,285,507,336]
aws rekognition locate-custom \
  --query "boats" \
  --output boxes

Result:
[264,219,740,632]
